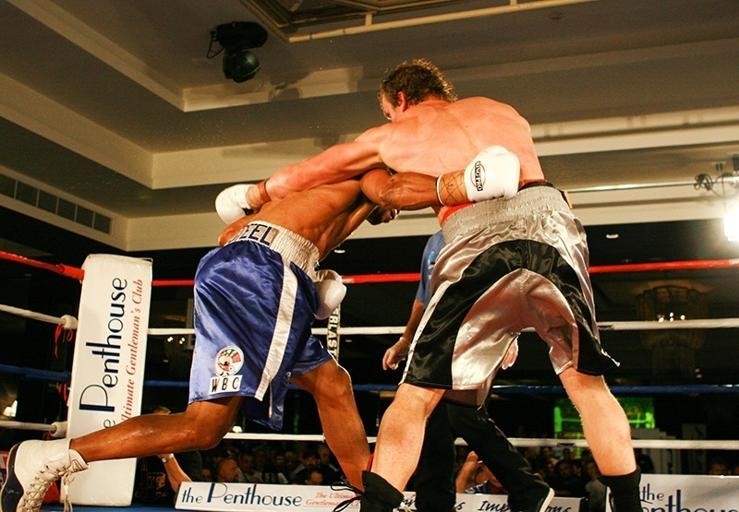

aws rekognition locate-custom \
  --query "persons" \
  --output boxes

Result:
[708,462,734,475]
[215,59,645,512]
[515,447,606,511]
[633,448,655,474]
[135,406,341,510]
[0,145,526,511]
[456,452,506,496]
[378,195,554,511]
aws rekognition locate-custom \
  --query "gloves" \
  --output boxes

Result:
[312,268,348,321]
[213,177,273,226]
[436,145,522,208]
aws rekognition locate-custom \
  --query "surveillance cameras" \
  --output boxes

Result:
[216,21,268,83]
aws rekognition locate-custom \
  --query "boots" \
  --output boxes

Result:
[0,437,89,511]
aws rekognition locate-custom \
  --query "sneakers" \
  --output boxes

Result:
[510,478,555,512]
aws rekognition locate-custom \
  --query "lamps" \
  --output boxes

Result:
[693,155,739,241]
